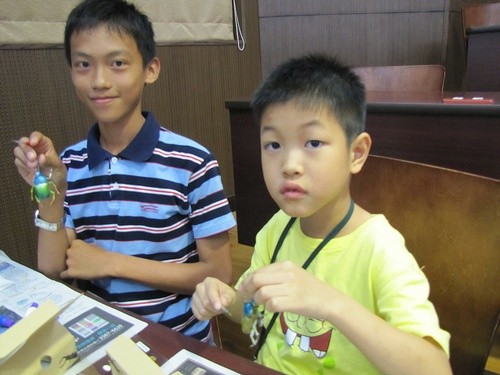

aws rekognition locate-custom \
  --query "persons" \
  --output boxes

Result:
[188,54,454,375]
[13,0,237,347]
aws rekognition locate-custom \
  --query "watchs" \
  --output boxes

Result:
[34,209,65,232]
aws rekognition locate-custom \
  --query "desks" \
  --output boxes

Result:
[224,98,500,249]
[49,278,286,375]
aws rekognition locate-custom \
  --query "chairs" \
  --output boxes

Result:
[351,154,500,375]
